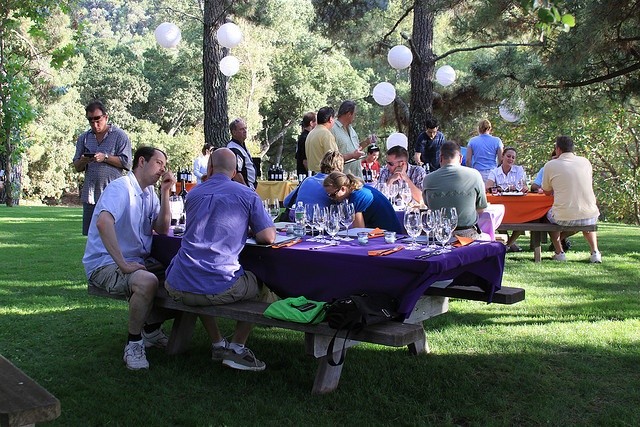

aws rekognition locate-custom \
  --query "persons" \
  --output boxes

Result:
[321,169,408,233]
[193,143,215,184]
[421,141,489,242]
[295,112,318,183]
[227,119,258,192]
[530,149,579,251]
[282,151,344,224]
[466,120,505,184]
[304,106,339,176]
[330,100,375,183]
[82,146,176,372]
[73,102,131,236]
[484,149,529,252]
[164,148,276,372]
[378,145,426,209]
[361,144,381,180]
[414,117,446,173]
[541,136,602,263]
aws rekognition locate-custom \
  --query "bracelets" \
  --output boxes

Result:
[104,154,109,162]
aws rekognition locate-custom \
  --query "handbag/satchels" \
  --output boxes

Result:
[262,295,328,325]
[323,295,401,366]
[274,208,295,223]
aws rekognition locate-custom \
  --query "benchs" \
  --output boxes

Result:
[497,222,598,263]
[1,353,61,426]
[87,283,424,396]
[422,280,525,316]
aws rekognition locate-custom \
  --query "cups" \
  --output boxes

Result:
[384,231,396,244]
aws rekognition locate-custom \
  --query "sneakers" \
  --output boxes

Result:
[548,243,555,251]
[222,348,266,371]
[511,243,522,251]
[561,239,571,251]
[552,251,565,260]
[123,338,149,371]
[141,326,169,349]
[211,337,230,361]
[589,251,602,263]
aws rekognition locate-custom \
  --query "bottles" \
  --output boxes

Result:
[423,162,430,174]
[267,163,283,180]
[177,165,192,183]
[367,163,372,182]
[178,178,188,223]
[169,183,178,225]
[362,163,367,183]
[294,201,304,235]
[298,169,317,185]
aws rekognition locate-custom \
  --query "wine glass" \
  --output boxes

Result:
[377,182,412,211]
[426,208,441,247]
[440,206,458,248]
[265,198,280,222]
[403,210,422,246]
[317,204,340,245]
[490,174,526,196]
[420,211,436,252]
[304,203,320,241]
[339,202,355,241]
[405,214,423,250]
[434,218,452,254]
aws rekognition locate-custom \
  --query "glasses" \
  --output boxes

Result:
[326,189,340,201]
[386,161,403,166]
[86,114,104,121]
[231,119,240,129]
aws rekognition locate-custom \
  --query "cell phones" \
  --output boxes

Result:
[84,153,96,159]
[290,302,317,312]
[393,165,404,179]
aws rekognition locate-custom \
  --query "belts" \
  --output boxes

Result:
[454,226,474,230]
